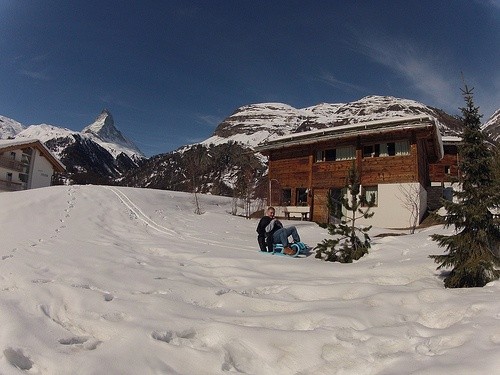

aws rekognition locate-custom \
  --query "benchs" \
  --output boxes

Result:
[282,210,309,221]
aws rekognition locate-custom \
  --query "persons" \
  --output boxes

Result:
[263,207,300,255]
